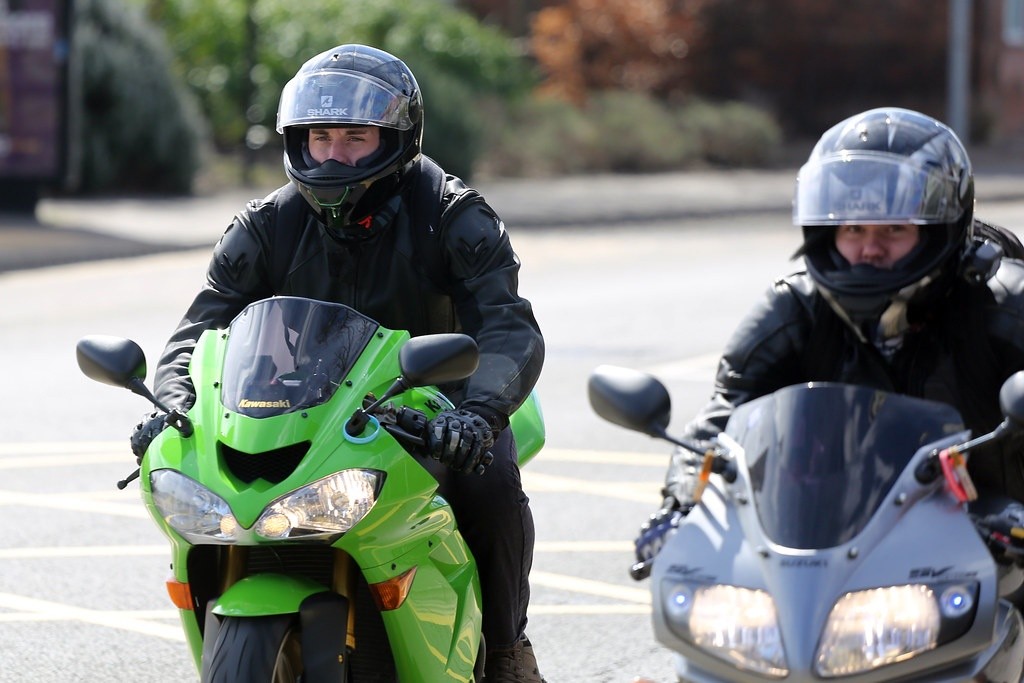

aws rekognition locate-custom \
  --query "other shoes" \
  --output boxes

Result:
[493,634,542,683]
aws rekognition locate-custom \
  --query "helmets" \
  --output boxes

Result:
[276,44,426,228]
[792,107,974,320]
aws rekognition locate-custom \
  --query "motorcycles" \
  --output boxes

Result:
[588,371,1024,683]
[76,296,545,683]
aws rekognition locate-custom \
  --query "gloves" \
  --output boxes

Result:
[131,415,167,459]
[634,509,681,565]
[426,407,493,475]
[986,502,1024,539]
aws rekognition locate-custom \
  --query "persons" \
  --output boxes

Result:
[634,108,1024,618]
[130,44,546,683]
